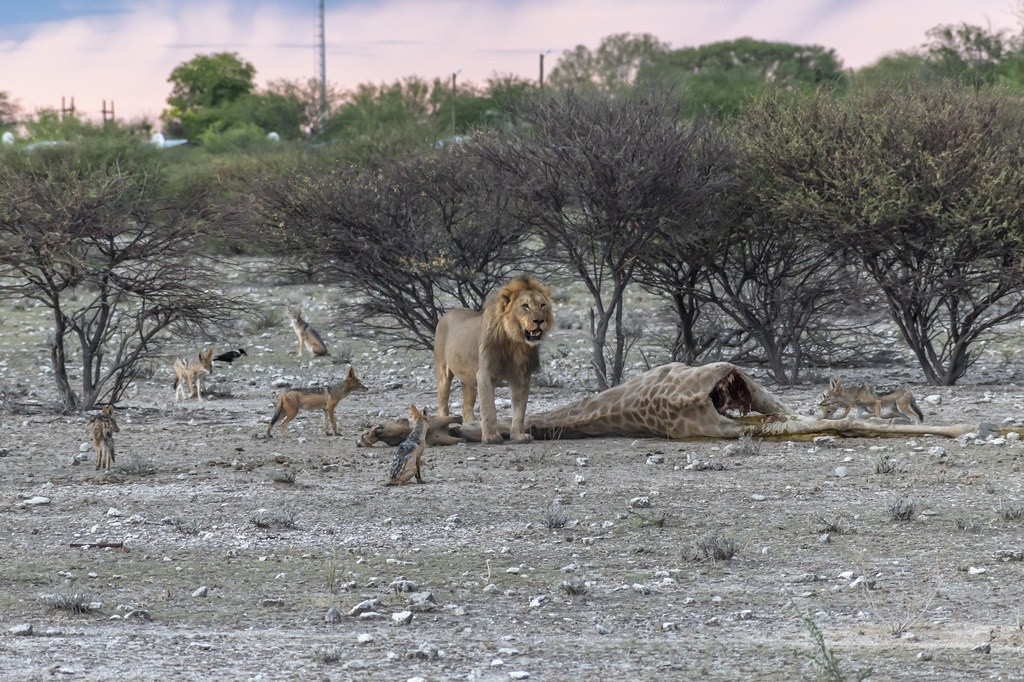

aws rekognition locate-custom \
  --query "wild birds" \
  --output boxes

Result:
[212,347,247,366]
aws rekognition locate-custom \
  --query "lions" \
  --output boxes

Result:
[431,274,554,447]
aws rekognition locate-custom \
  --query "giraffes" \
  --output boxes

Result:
[357,361,1002,448]
